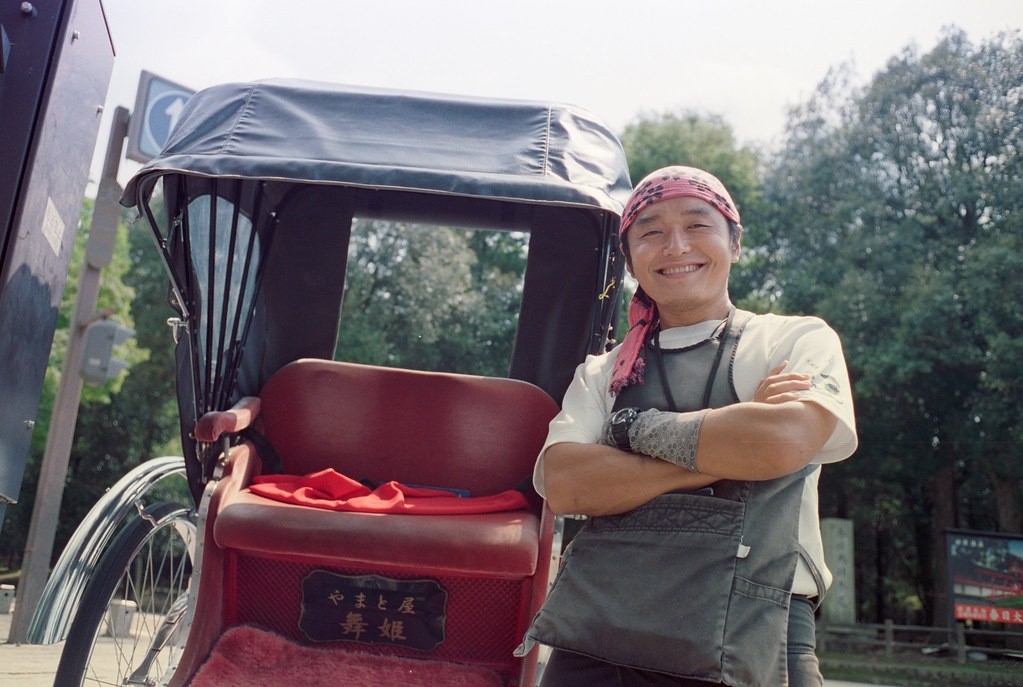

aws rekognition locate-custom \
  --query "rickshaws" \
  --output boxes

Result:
[29,78,637,687]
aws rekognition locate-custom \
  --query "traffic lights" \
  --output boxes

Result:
[77,319,136,383]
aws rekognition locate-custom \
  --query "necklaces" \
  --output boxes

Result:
[652,304,737,415]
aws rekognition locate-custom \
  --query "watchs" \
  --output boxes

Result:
[610,406,641,453]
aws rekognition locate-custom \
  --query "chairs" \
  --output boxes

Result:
[167,354,564,687]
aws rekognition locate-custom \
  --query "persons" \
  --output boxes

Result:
[527,164,860,687]
